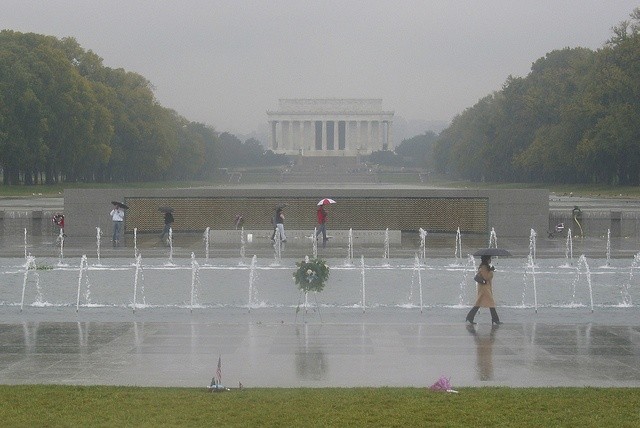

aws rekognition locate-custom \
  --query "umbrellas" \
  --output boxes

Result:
[111,200,130,209]
[473,248,513,268]
[271,203,289,211]
[317,198,336,206]
[157,206,174,212]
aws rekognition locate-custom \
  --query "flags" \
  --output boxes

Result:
[210,357,222,387]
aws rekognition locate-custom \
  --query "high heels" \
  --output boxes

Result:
[465,318,477,324]
[492,320,504,324]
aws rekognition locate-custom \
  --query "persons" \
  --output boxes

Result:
[312,204,329,240]
[109,204,125,244]
[273,209,288,242]
[52,211,64,229]
[572,206,583,237]
[270,209,284,241]
[160,211,175,239]
[465,255,503,325]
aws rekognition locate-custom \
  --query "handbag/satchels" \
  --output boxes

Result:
[474,271,486,284]
